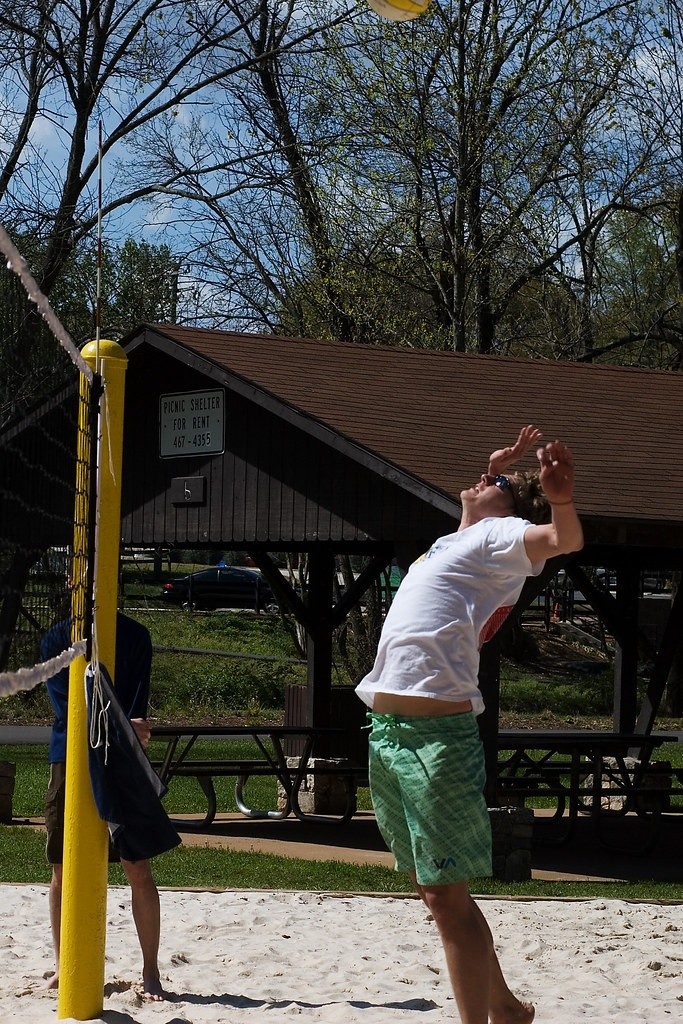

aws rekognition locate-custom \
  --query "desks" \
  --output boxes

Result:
[148,725,360,824]
[497,730,678,843]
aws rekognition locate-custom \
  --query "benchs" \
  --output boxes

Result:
[151,757,683,795]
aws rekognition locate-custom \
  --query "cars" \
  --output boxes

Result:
[162,567,280,615]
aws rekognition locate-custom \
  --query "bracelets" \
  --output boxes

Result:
[549,496,574,505]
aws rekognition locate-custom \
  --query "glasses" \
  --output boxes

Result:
[495,474,517,504]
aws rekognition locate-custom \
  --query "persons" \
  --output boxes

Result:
[43,564,183,1003]
[353,424,584,1023]
